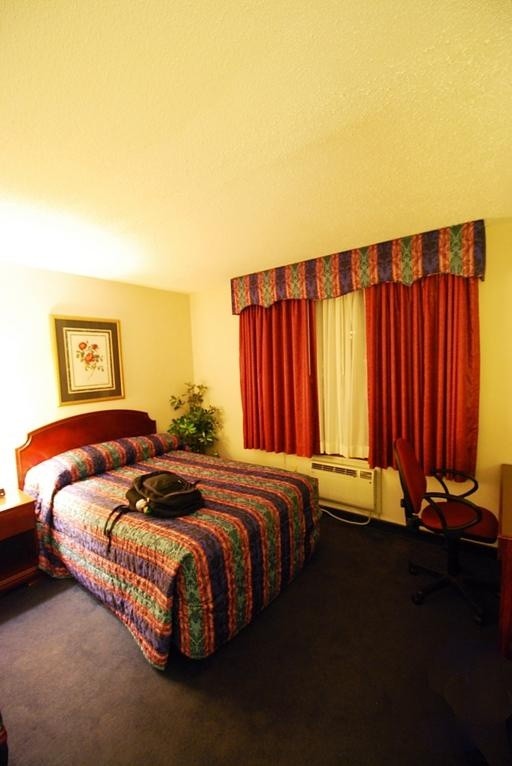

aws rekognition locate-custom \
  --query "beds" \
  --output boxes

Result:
[14,407,319,671]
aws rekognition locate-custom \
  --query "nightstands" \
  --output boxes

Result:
[0,486,41,596]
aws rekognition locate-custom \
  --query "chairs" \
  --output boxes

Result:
[391,437,499,624]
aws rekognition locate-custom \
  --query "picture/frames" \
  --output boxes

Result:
[48,313,126,408]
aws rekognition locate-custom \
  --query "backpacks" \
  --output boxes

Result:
[125,470,208,520]
